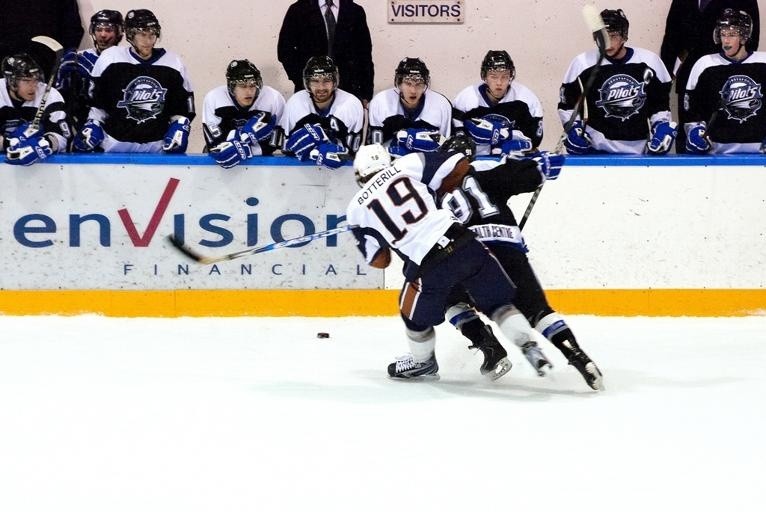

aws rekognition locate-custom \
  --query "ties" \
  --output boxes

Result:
[324,0,340,68]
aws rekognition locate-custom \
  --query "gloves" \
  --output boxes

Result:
[225,110,278,147]
[682,120,713,154]
[285,121,329,162]
[208,139,254,170]
[6,120,57,167]
[646,117,679,156]
[160,114,192,155]
[561,120,594,156]
[308,142,351,171]
[462,115,513,144]
[70,118,107,154]
[395,127,447,154]
[529,150,566,182]
[500,129,533,158]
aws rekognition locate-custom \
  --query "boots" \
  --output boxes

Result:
[557,336,604,391]
[467,324,513,384]
[518,340,555,378]
[386,348,440,384]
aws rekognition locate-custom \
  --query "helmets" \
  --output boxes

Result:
[480,49,517,86]
[1,51,45,81]
[123,8,162,40]
[591,8,631,46]
[225,57,264,97]
[352,141,392,190]
[302,54,341,95]
[439,135,476,163]
[394,55,432,94]
[88,8,125,44]
[712,6,754,47]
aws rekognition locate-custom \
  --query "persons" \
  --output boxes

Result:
[679,6,766,156]
[1,3,85,86]
[366,55,452,159]
[200,59,287,171]
[556,9,679,156]
[428,133,603,391]
[276,0,375,115]
[71,8,196,157]
[344,143,555,378]
[278,52,366,171]
[659,1,760,155]
[453,50,545,155]
[54,8,126,153]
[1,50,72,169]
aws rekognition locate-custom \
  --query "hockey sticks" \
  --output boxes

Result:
[515,5,611,233]
[165,226,350,266]
[30,36,64,138]
